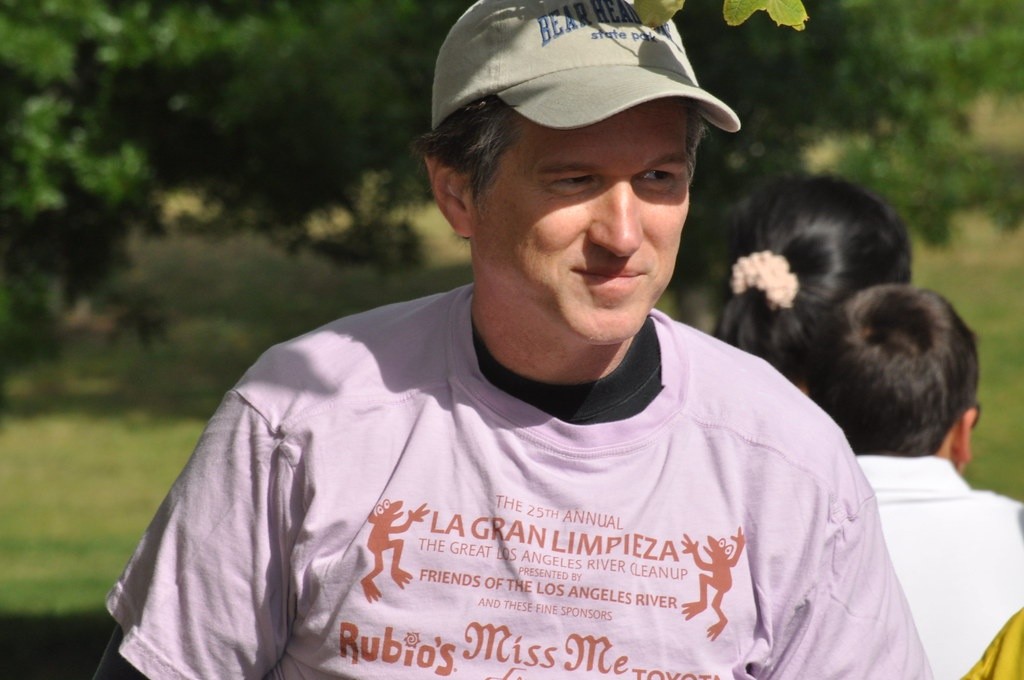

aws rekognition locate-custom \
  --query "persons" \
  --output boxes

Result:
[92,0,938,680]
[809,282,1024,680]
[712,174,911,396]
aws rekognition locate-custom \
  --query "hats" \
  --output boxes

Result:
[432,0,742,134]
